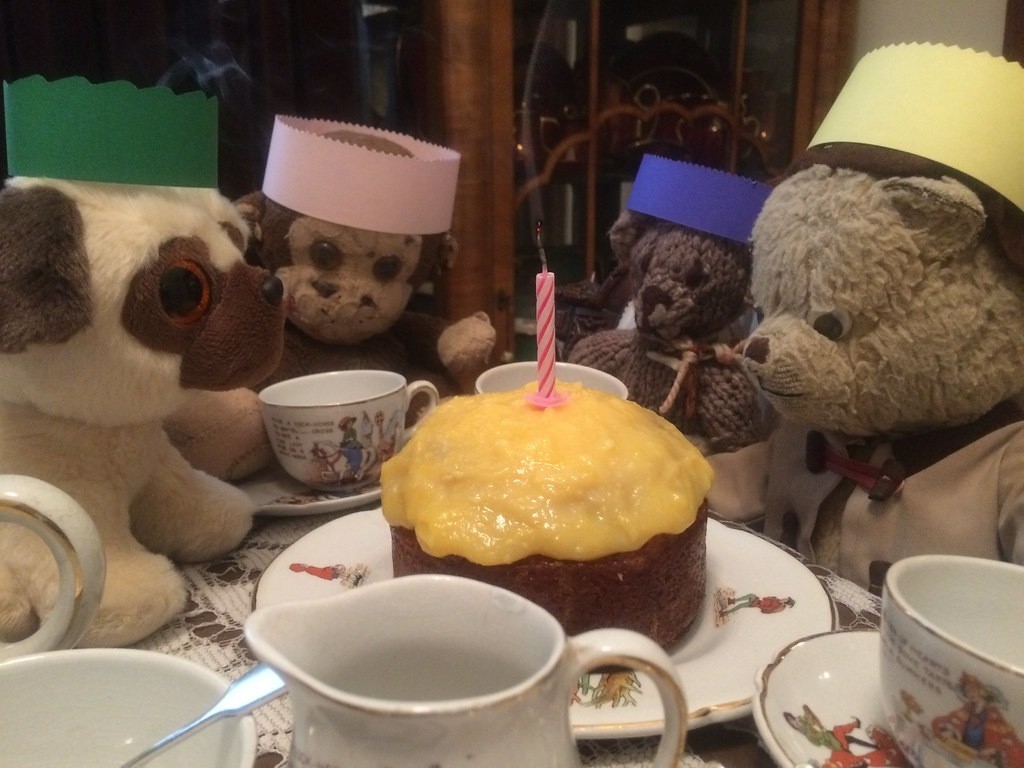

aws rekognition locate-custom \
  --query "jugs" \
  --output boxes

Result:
[244,573,688,768]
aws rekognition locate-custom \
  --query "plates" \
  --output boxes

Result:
[251,507,840,740]
[750,627,912,768]
[234,474,383,518]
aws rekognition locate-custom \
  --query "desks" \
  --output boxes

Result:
[128,499,888,768]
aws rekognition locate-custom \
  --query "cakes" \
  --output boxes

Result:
[379,379,715,671]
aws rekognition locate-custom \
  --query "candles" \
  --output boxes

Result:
[527,220,568,407]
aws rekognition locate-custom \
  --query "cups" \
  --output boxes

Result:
[878,553,1024,768]
[0,643,257,768]
[473,360,629,401]
[257,369,440,492]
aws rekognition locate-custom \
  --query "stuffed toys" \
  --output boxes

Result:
[566,209,773,459]
[0,175,291,646]
[741,144,1023,596]
[228,130,500,428]
